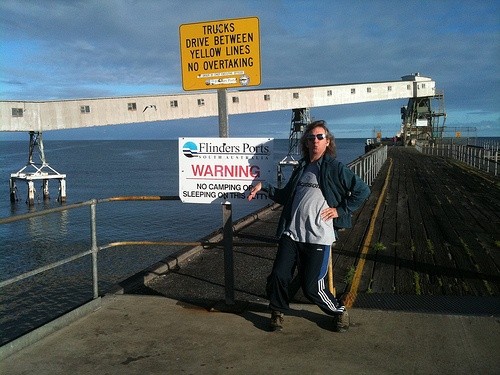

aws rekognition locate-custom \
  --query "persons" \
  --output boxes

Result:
[247,120,370,331]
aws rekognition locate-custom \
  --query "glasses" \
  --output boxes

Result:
[307,133,327,141]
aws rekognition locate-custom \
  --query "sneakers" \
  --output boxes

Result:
[337,309,349,333]
[271,310,284,331]
